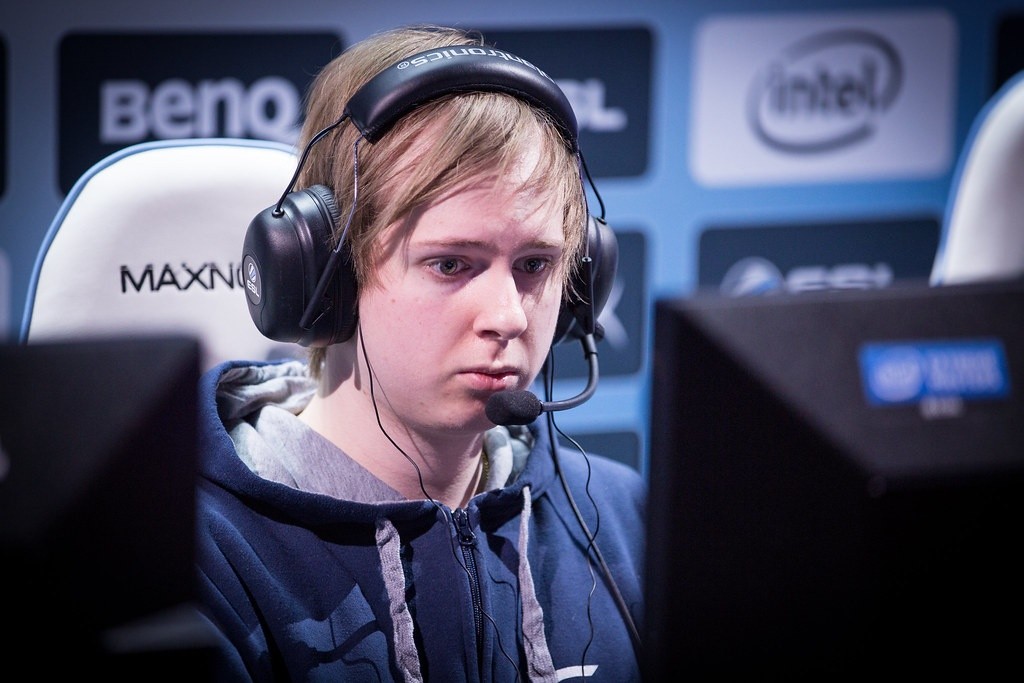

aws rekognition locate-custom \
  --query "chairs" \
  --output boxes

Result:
[19,132,334,377]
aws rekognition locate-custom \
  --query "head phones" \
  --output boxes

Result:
[242,46,619,349]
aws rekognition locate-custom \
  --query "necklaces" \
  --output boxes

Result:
[479,451,490,492]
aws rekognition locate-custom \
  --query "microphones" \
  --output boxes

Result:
[485,322,606,427]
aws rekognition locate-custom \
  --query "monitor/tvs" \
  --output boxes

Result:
[0,333,207,683]
[635,274,1024,683]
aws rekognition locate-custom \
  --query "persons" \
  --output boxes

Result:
[193,24,646,683]
